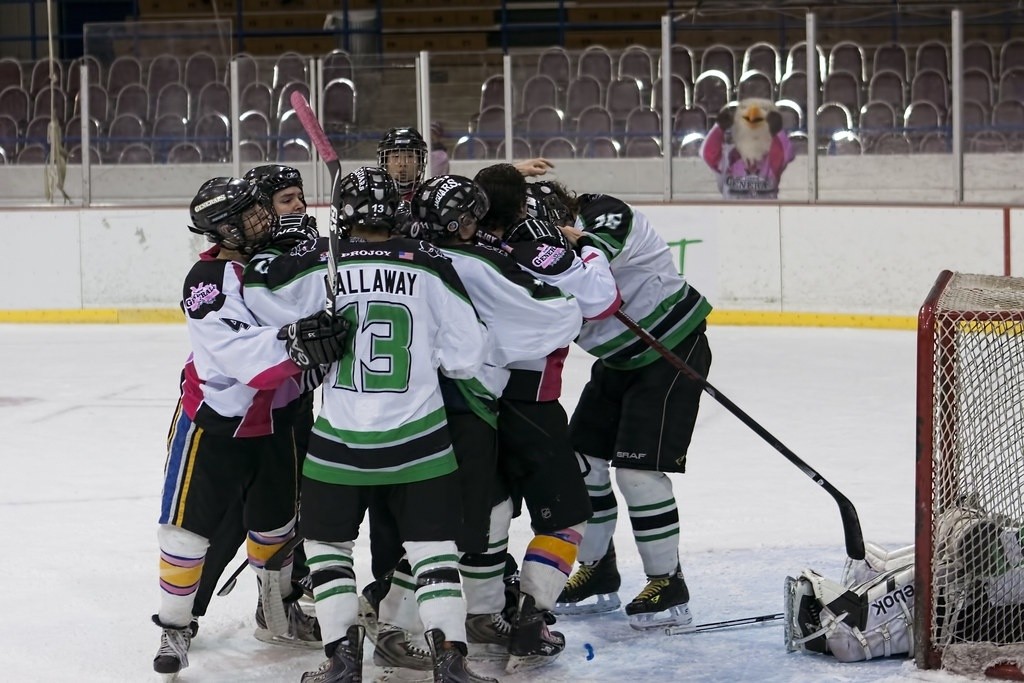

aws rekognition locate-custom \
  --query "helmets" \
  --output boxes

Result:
[189,177,263,242]
[412,174,490,243]
[243,165,304,211]
[526,181,570,226]
[338,165,400,227]
[379,126,427,165]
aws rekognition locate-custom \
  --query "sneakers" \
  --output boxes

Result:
[784,576,827,654]
[254,575,324,649]
[190,615,200,638]
[294,570,313,601]
[297,625,366,683]
[497,551,522,623]
[151,613,193,683]
[503,590,565,674]
[464,613,512,662]
[422,629,499,683]
[625,548,693,631]
[357,569,394,645]
[372,623,435,683]
[551,536,621,614]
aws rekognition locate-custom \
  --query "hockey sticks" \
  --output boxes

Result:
[665,612,784,636]
[615,308,865,560]
[291,90,342,317]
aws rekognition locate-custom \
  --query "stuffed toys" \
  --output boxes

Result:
[699,97,796,200]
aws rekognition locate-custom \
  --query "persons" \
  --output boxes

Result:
[151,128,712,683]
[785,487,1024,663]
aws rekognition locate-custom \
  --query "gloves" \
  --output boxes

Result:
[271,213,318,250]
[277,310,353,371]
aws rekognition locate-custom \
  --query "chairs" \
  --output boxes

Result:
[146,53,181,105]
[275,80,311,125]
[450,37,1024,160]
[0,57,23,94]
[196,80,231,122]
[323,78,358,124]
[65,56,102,106]
[113,83,149,127]
[0,85,30,126]
[33,85,67,130]
[72,84,109,130]
[29,57,63,99]
[272,50,309,93]
[105,57,142,100]
[154,81,192,126]
[183,50,217,113]
[322,49,354,90]
[239,81,273,122]
[0,108,312,164]
[224,52,258,98]
